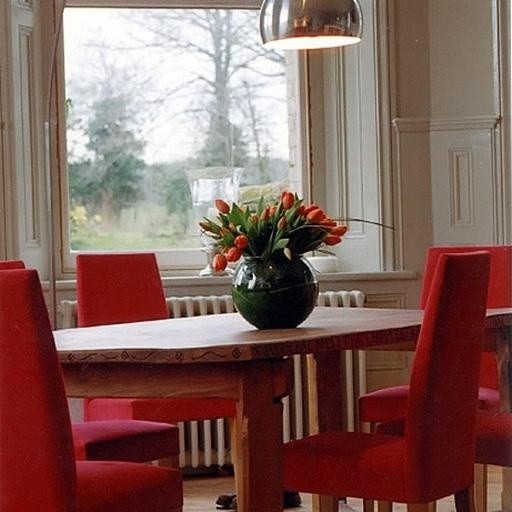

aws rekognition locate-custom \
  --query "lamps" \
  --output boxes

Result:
[258,0,361,53]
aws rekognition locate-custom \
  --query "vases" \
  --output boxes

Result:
[233,256,317,328]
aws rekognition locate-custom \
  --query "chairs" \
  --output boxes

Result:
[74,250,258,464]
[261,250,495,508]
[356,247,510,505]
[1,258,185,505]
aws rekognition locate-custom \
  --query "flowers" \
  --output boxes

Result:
[199,188,396,275]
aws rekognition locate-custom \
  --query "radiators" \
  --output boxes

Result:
[65,296,360,480]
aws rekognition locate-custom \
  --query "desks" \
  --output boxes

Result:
[50,293,505,502]
